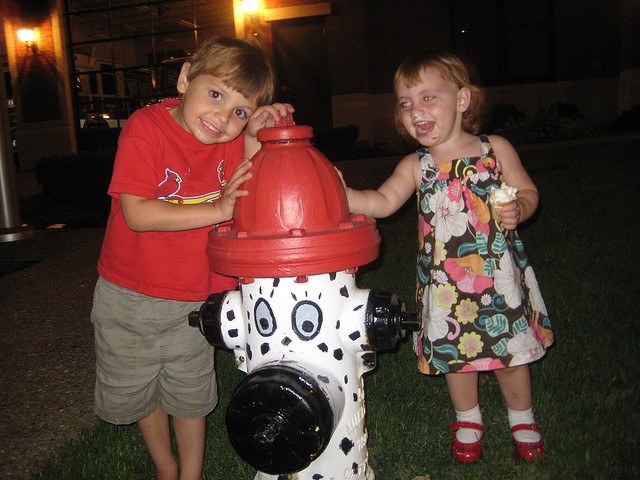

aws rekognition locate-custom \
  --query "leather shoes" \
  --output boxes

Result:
[509,422,545,462]
[448,419,485,463]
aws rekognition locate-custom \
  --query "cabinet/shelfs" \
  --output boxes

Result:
[74,53,127,101]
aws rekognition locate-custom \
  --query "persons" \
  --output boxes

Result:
[334,48,553,463]
[90,36,296,480]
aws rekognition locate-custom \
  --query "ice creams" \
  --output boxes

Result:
[489,182,518,221]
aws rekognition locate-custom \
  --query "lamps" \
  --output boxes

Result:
[14,18,38,53]
[242,1,261,39]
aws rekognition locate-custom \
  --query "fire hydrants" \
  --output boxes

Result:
[187,110,420,479]
